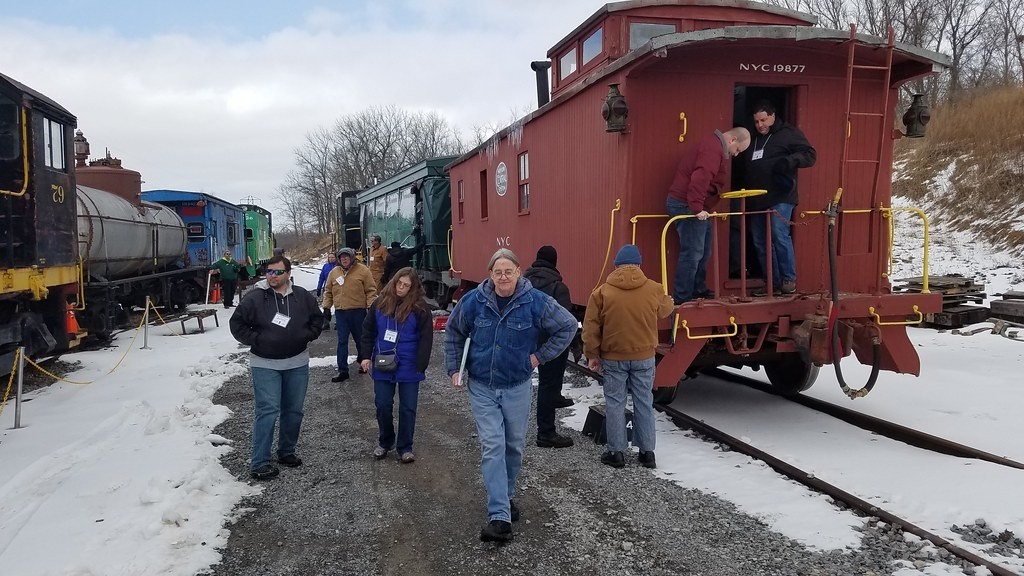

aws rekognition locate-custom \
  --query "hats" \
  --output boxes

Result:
[614,244,641,265]
[536,246,557,267]
[391,242,400,248]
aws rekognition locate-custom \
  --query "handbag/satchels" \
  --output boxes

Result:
[374,355,400,373]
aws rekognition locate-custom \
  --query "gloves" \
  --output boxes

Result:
[323,308,332,320]
[773,158,789,175]
[317,289,322,297]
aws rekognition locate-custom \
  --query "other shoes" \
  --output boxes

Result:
[601,451,625,468]
[536,433,574,448]
[699,289,720,299]
[555,395,575,408]
[332,372,349,382]
[638,449,656,467]
[481,519,513,543]
[510,500,520,521]
[373,444,395,459]
[224,305,237,309]
[250,465,279,479]
[333,323,337,330]
[358,366,367,374]
[277,454,302,468]
[402,452,414,463]
[322,323,330,330]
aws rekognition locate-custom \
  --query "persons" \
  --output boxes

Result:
[317,252,342,330]
[443,247,578,540]
[208,250,241,309]
[378,236,426,289]
[746,102,817,298]
[321,247,378,382]
[412,201,424,268]
[359,267,433,462]
[582,244,675,467]
[522,245,574,447]
[666,127,752,305]
[229,256,324,479]
[367,235,388,288]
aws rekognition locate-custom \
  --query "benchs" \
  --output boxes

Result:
[181,306,218,334]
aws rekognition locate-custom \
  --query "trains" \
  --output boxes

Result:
[1,74,275,373]
[329,0,955,407]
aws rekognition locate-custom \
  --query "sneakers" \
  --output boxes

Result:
[753,285,781,296]
[782,280,796,293]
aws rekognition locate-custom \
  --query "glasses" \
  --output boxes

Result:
[267,269,287,276]
[491,267,517,280]
